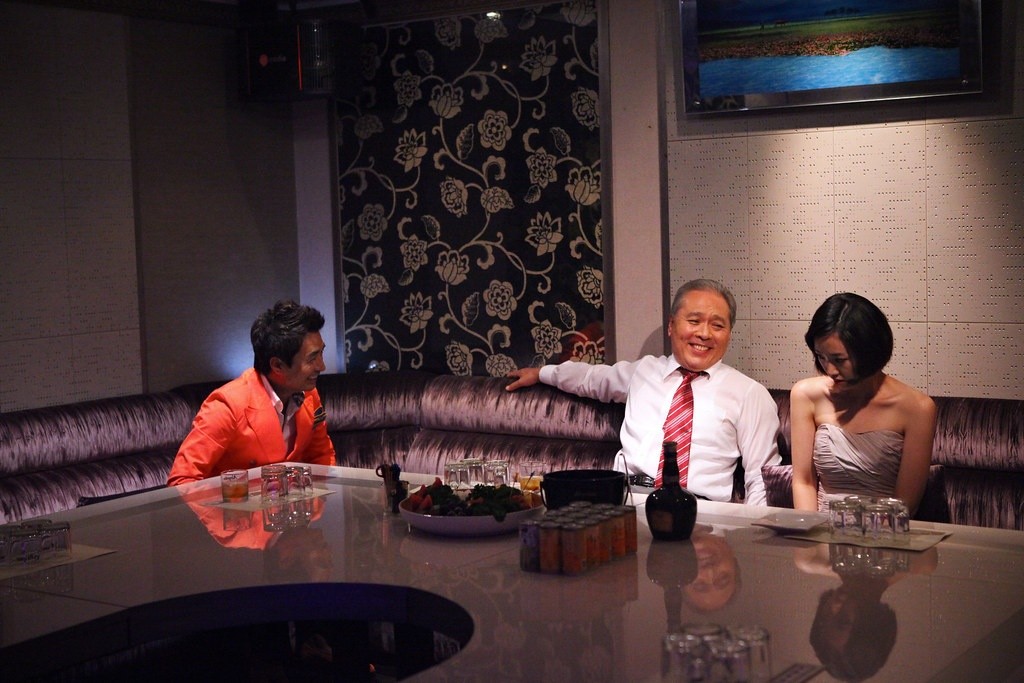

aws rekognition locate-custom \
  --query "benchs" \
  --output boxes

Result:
[0,372,1023,531]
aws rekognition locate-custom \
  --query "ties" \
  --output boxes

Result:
[656,365,701,490]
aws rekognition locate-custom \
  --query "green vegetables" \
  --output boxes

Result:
[423,484,521,523]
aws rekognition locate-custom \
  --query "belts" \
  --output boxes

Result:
[628,475,655,488]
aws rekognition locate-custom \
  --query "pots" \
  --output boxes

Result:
[543,469,629,512]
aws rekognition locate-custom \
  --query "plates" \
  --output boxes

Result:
[398,492,545,537]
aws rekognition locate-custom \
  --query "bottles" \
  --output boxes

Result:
[645,441,698,541]
[646,538,698,624]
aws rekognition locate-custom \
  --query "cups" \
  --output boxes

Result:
[661,622,772,683]
[220,465,313,503]
[830,544,910,578]
[828,495,909,544]
[0,520,73,569]
[2,564,73,602]
[223,498,314,532]
[444,458,547,496]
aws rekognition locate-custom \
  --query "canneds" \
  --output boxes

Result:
[518,501,637,577]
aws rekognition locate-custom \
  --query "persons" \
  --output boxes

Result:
[791,293,936,521]
[791,522,938,682]
[507,278,780,508]
[626,493,778,615]
[167,300,336,487]
[179,477,332,585]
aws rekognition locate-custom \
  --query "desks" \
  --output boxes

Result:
[0,456,1024,683]
